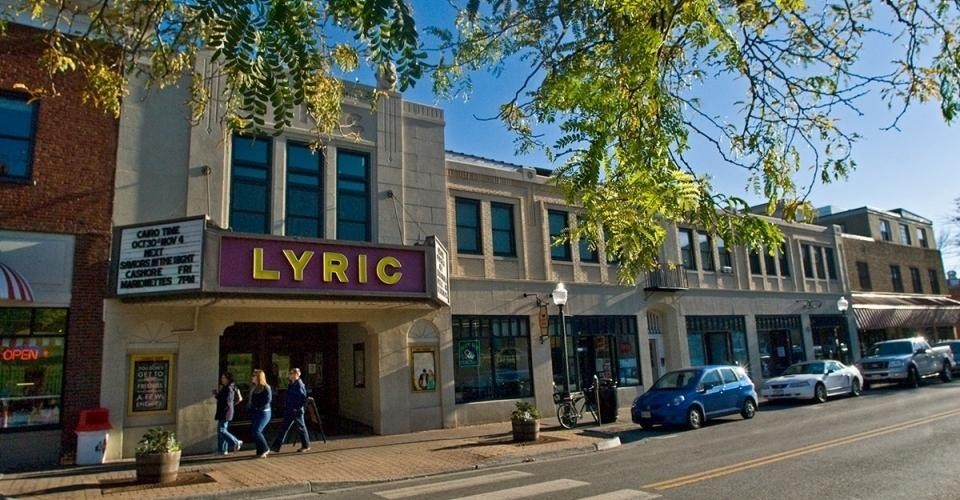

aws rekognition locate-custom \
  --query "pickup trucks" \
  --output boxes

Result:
[857,337,955,388]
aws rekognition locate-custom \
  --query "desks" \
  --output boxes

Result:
[0,395,61,429]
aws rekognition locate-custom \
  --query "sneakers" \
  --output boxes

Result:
[221,440,313,460]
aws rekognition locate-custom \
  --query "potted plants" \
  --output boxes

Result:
[134,426,184,484]
[511,401,542,442]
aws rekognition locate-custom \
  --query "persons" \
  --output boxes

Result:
[270,366,311,453]
[245,368,272,459]
[211,369,244,455]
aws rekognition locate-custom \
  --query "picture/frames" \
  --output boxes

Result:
[458,340,480,368]
[410,345,438,393]
[353,342,365,389]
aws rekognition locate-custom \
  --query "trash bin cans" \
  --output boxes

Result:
[598,378,618,424]
[73,408,113,465]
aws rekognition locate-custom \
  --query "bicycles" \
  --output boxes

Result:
[557,392,599,429]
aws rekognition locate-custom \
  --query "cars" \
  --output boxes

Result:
[631,365,758,429]
[761,359,864,403]
[936,339,960,380]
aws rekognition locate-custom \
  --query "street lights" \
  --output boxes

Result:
[552,284,568,416]
